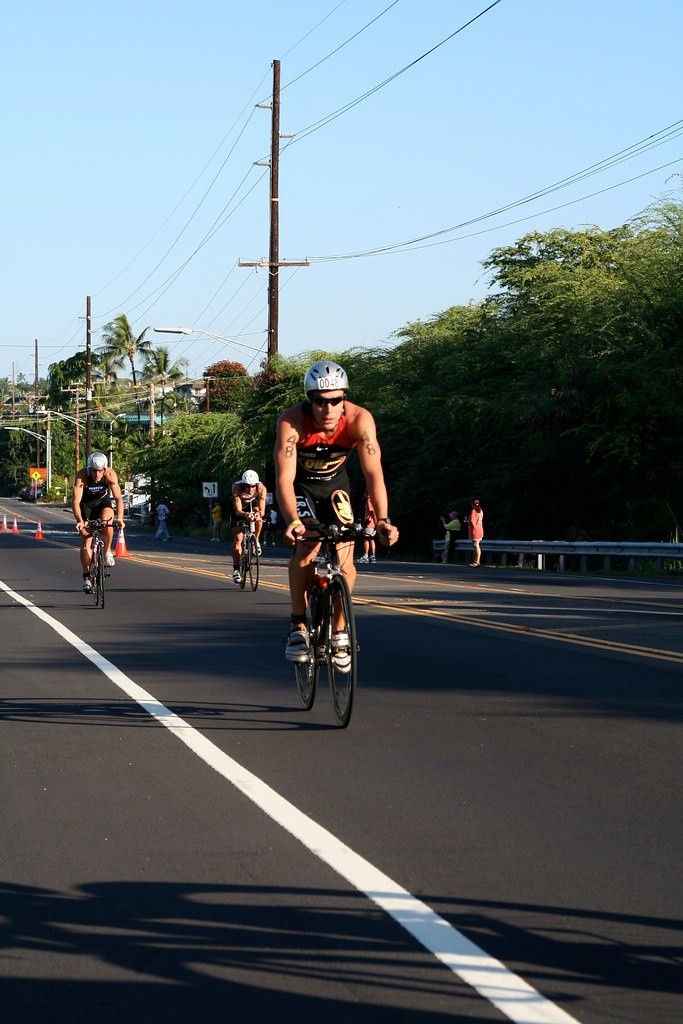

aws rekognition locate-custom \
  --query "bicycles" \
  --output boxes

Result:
[79,517,122,610]
[236,513,266,592]
[281,522,391,729]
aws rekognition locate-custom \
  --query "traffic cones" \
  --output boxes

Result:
[63,496,67,504]
[0,514,9,532]
[32,519,45,540]
[12,516,19,534]
[113,525,132,557]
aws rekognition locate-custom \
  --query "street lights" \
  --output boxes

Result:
[36,410,91,467]
[152,326,278,531]
[109,413,127,499]
[4,426,51,493]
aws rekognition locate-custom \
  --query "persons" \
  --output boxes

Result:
[441,512,461,564]
[209,501,222,542]
[72,452,126,591]
[356,487,376,563]
[155,500,173,541]
[274,361,399,673]
[464,499,484,567]
[231,470,267,584]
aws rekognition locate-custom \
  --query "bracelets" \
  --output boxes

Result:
[257,510,262,518]
[291,520,302,525]
[245,512,249,517]
[117,518,124,521]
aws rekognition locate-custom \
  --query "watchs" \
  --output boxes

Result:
[376,517,393,524]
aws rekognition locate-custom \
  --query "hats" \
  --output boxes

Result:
[449,511,458,519]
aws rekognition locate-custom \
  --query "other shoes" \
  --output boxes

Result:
[210,538,220,543]
[469,561,481,567]
[356,556,369,564]
[369,556,377,564]
[441,561,447,564]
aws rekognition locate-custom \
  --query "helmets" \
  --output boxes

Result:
[304,360,349,396]
[242,470,260,485]
[87,452,108,472]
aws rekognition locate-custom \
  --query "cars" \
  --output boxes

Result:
[18,487,45,502]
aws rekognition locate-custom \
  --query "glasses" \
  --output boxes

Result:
[245,484,256,488]
[91,468,104,472]
[311,394,346,406]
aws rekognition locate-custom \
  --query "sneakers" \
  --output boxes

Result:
[103,552,115,567]
[331,633,352,672]
[82,579,92,590]
[233,570,241,583]
[285,627,310,663]
[252,541,261,556]
